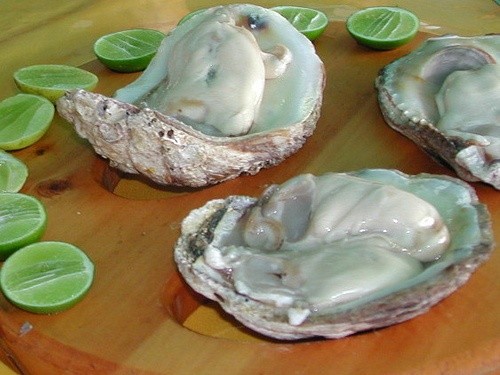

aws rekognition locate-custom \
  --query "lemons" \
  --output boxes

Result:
[94,29,168,73]
[1,149,30,194]
[346,6,420,51]
[1,94,56,150]
[177,8,210,27]
[1,192,48,262]
[1,241,97,315]
[13,64,98,104]
[268,5,330,42]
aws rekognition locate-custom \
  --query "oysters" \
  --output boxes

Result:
[171,169,494,339]
[374,32,500,191]
[57,2,326,188]
